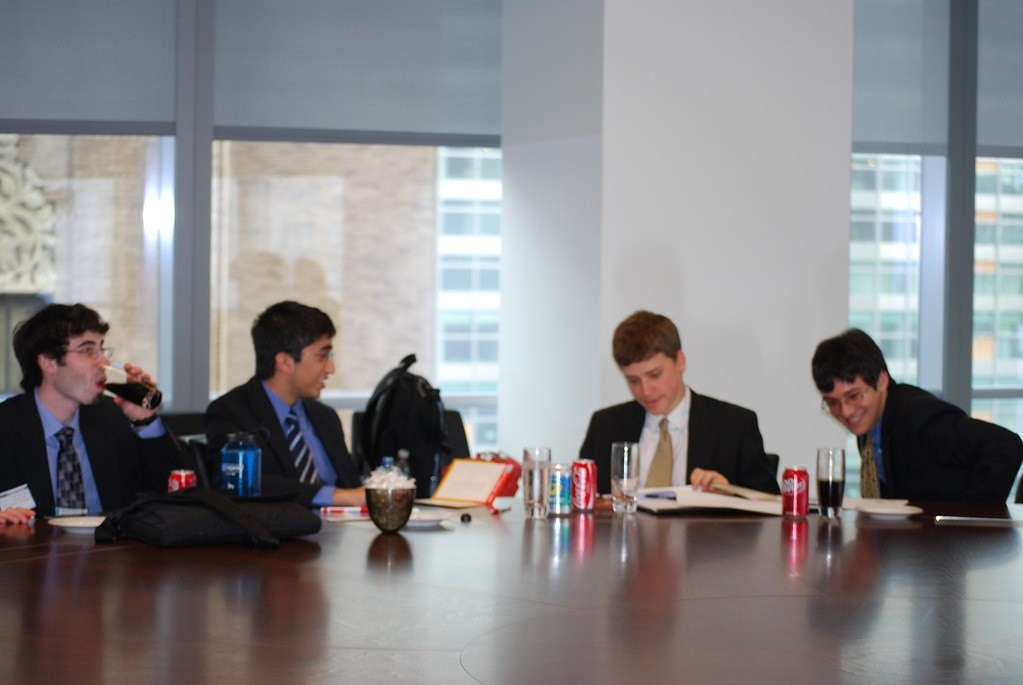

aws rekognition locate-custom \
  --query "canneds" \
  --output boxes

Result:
[781,467,809,519]
[548,459,597,514]
[167,469,196,493]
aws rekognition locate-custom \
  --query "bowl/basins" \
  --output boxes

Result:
[364,488,415,532]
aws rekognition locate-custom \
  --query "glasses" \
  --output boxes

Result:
[51,346,112,363]
[821,383,871,413]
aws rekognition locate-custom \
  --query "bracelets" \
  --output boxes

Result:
[132,414,157,426]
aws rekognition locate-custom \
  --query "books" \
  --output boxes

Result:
[638,483,784,514]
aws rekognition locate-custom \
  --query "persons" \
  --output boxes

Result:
[1,303,183,523]
[206,301,370,506]
[811,329,1023,517]
[580,312,780,497]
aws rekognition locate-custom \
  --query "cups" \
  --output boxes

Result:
[522,446,550,519]
[817,448,845,517]
[99,363,162,410]
[611,442,639,513]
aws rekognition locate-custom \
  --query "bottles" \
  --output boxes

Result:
[219,432,262,499]
[382,450,410,473]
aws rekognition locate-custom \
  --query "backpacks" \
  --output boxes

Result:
[355,354,443,499]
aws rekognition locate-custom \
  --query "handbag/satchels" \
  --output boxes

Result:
[94,486,321,547]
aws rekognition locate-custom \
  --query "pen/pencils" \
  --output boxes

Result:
[488,505,511,515]
[642,492,676,501]
[320,505,368,513]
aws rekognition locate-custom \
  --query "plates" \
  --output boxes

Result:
[48,516,104,534]
[406,510,448,526]
[855,502,922,520]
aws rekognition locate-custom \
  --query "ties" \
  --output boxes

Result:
[646,418,672,487]
[861,433,880,499]
[53,427,85,509]
[285,410,321,487]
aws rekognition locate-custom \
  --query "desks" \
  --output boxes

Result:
[0,507,1023,685]
